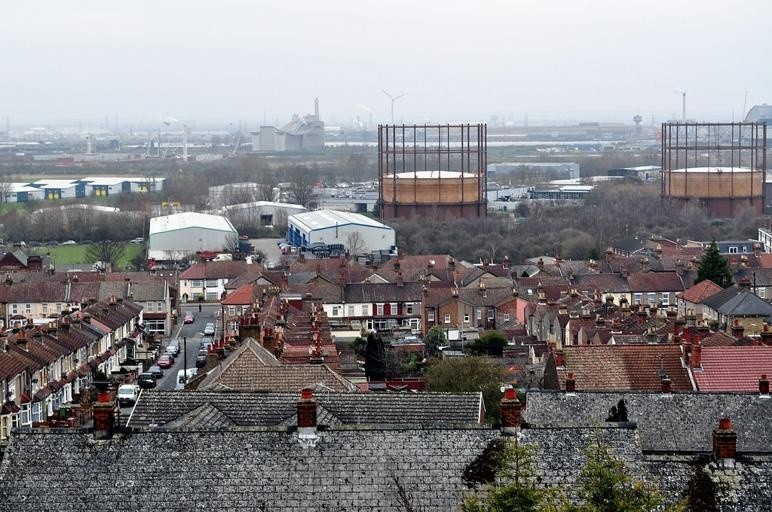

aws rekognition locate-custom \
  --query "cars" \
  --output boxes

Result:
[196,337,213,366]
[148,365,163,377]
[164,340,180,356]
[183,311,195,324]
[204,322,215,334]
[157,353,174,369]
[177,368,197,389]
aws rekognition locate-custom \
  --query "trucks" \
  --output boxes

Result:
[116,383,140,405]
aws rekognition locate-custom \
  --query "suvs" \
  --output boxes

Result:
[138,373,156,388]
